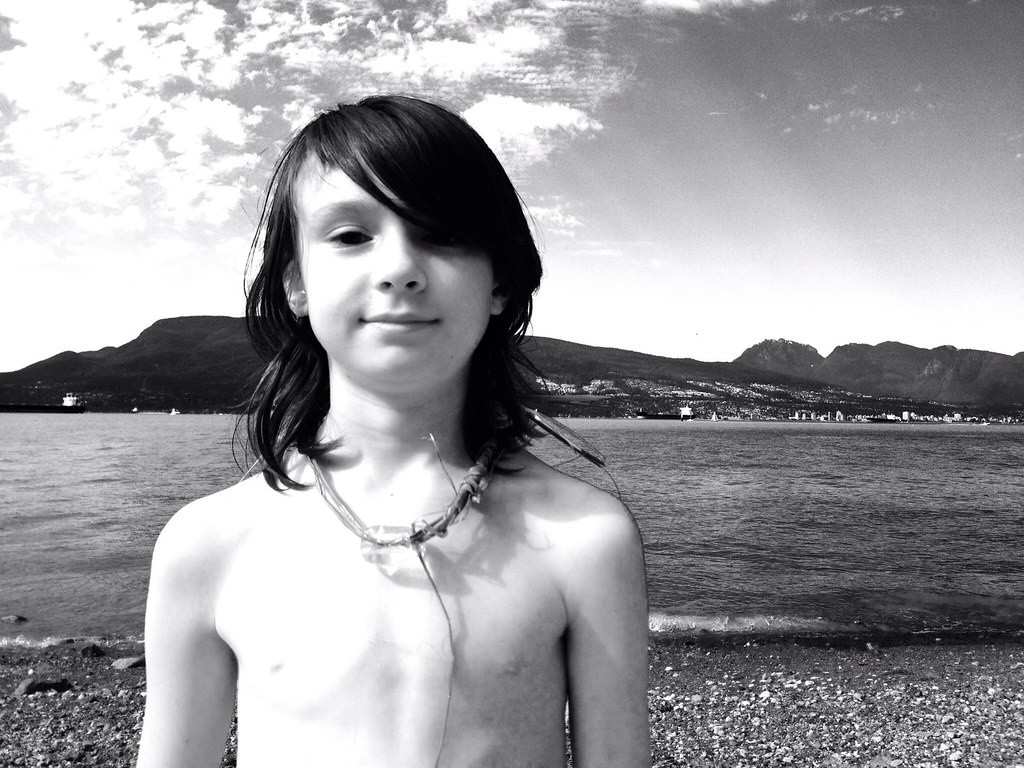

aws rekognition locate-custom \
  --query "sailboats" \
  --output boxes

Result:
[710,412,718,422]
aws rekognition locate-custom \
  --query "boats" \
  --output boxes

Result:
[0,392,88,414]
[131,406,139,413]
[170,408,180,416]
[687,418,693,421]
[866,413,900,423]
[970,419,990,426]
[636,404,696,420]
[724,414,744,421]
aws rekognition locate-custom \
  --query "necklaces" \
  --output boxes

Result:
[305,431,495,768]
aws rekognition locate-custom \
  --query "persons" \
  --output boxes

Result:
[135,96,650,768]
